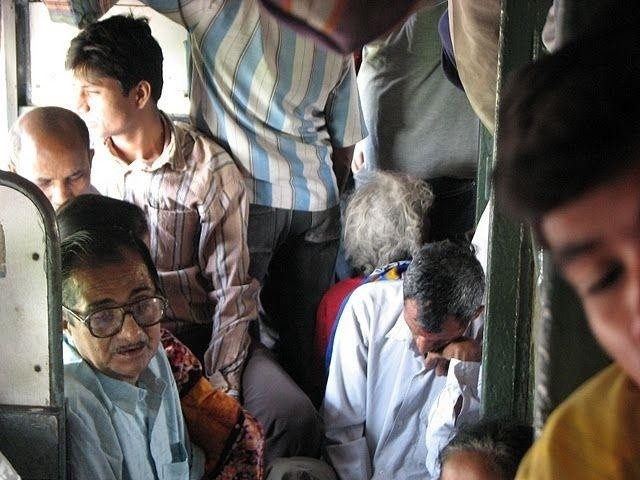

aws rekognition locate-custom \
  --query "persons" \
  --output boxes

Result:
[309,166,439,367]
[435,415,535,480]
[320,235,487,480]
[58,190,265,479]
[55,226,210,479]
[49,1,372,409]
[346,4,483,259]
[6,104,103,220]
[64,7,329,478]
[509,25,640,480]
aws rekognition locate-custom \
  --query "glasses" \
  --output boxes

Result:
[61,295,168,339]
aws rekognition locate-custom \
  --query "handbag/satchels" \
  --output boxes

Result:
[157,327,267,480]
